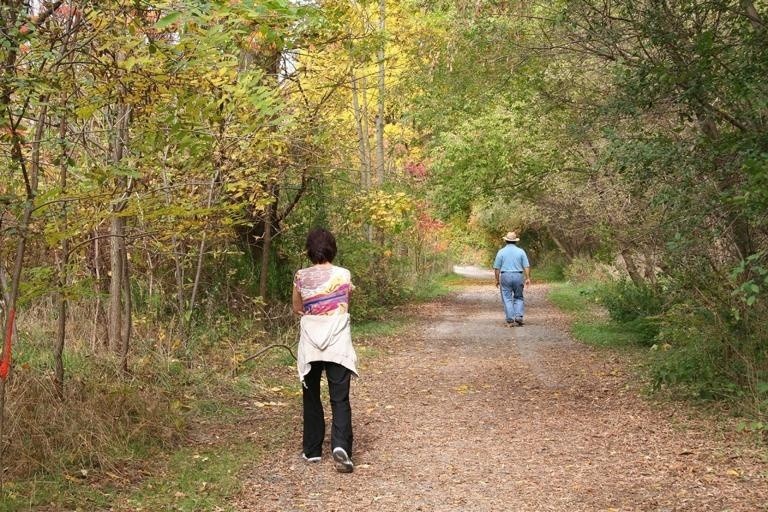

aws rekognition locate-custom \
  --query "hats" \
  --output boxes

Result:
[502,232,520,241]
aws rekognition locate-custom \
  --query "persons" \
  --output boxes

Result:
[493,232,531,328]
[291,227,360,474]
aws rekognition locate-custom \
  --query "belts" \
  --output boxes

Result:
[500,271,524,273]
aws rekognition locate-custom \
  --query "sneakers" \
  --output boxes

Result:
[302,453,322,463]
[508,319,524,327]
[332,447,353,473]
